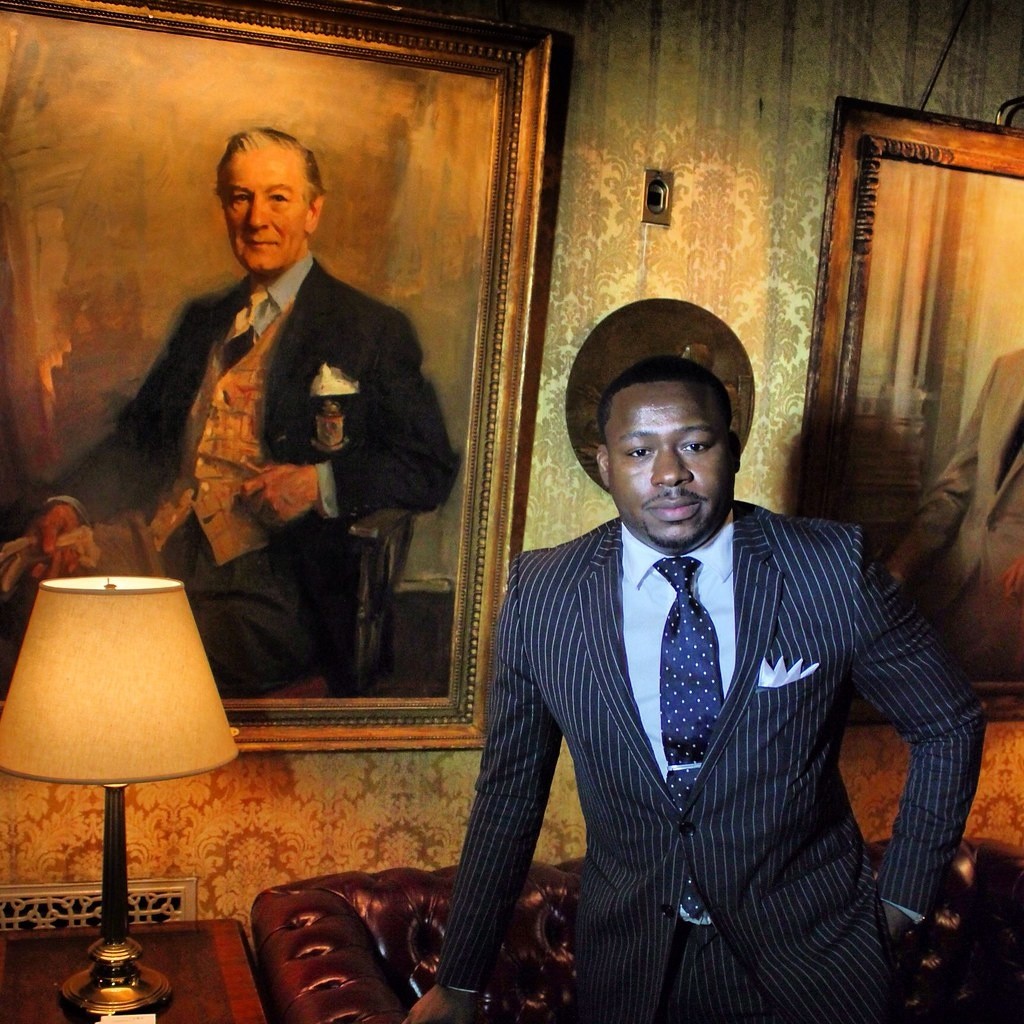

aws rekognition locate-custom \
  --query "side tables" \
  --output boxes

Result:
[0,917,267,1024]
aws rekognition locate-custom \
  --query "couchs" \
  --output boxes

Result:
[252,832,1024,1023]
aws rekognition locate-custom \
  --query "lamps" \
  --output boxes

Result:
[1,574,236,1021]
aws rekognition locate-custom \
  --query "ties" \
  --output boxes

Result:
[653,557,726,922]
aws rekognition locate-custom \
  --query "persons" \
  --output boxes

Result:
[397,356,994,1024]
[27,129,461,696]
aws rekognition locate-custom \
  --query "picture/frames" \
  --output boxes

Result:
[0,1,565,754]
[799,86,1022,725]
